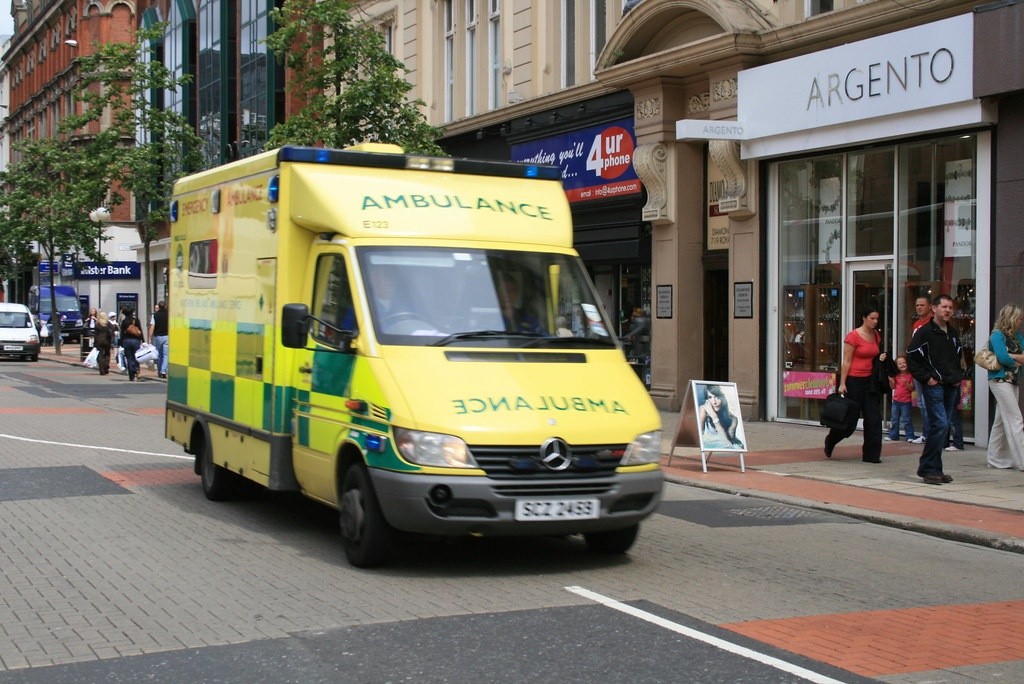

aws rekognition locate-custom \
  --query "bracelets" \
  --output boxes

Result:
[712,419,720,424]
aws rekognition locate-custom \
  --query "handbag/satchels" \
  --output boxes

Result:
[115,346,127,371]
[83,322,89,328]
[820,393,859,430]
[127,320,141,336]
[973,330,1006,370]
[135,342,159,361]
[84,346,100,367]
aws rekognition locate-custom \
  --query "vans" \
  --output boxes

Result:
[29,286,83,344]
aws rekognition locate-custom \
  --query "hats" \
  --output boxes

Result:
[109,312,116,317]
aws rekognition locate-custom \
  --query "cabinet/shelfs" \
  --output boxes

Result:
[903,281,975,420]
[782,284,842,422]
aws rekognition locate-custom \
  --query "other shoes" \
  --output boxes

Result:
[136,370,142,378]
[885,437,891,440]
[945,446,959,450]
[908,439,913,442]
[917,470,943,483]
[942,475,953,482]
[912,436,926,443]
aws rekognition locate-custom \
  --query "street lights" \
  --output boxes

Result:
[89,208,110,312]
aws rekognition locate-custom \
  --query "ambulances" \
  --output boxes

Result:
[163,143,665,570]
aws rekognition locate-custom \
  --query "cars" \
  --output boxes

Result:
[0,303,41,362]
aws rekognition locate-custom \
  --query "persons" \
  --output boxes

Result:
[699,384,744,449]
[84,301,168,381]
[824,293,1024,484]
[478,275,549,337]
[951,279,976,379]
[342,270,400,333]
[47,308,64,346]
